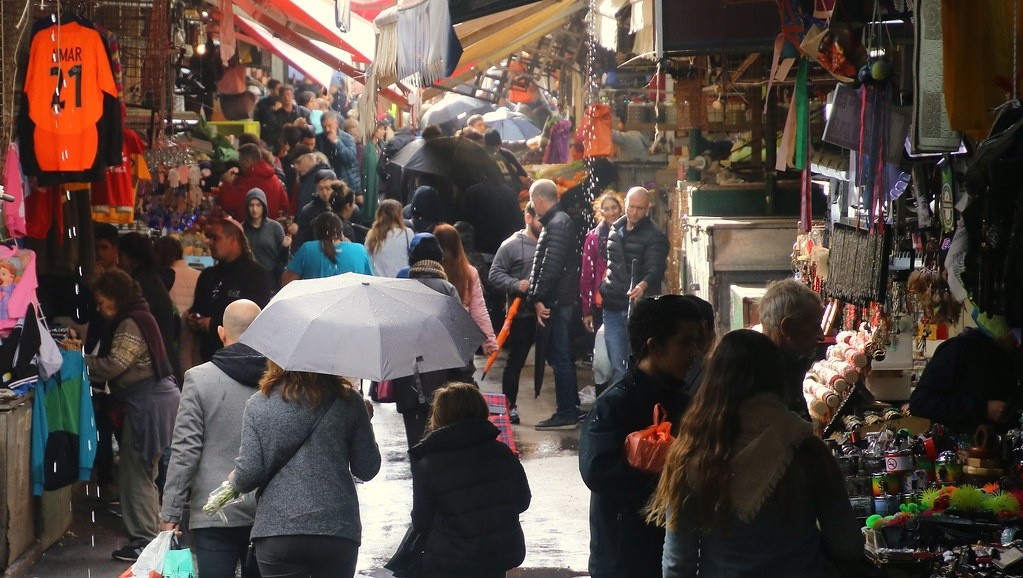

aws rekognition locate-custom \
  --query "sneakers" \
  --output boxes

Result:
[112,545,145,560]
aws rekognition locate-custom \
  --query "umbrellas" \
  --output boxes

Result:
[387,133,506,184]
[420,90,490,132]
[482,107,542,140]
[235,271,488,400]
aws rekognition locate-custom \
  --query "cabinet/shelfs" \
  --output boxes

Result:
[0,392,36,569]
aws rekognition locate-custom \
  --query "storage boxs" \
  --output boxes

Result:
[917,324,938,340]
[967,457,995,468]
[860,416,932,442]
[864,370,914,402]
[870,333,916,369]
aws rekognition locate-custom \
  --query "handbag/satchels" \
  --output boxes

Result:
[624,402,675,472]
[117,529,196,578]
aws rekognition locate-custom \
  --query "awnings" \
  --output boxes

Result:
[363,0,591,91]
[218,0,412,113]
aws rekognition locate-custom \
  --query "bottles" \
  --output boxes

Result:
[598,92,827,133]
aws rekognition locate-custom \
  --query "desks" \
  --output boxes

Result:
[724,283,767,331]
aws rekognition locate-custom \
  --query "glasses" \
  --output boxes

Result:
[291,156,306,167]
[600,207,619,213]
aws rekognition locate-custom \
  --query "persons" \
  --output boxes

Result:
[387,382,531,578]
[760,281,873,417]
[640,330,869,578]
[578,293,716,577]
[86,62,670,515]
[231,360,382,578]
[81,271,181,564]
[160,300,262,578]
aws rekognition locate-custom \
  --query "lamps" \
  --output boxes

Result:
[180,42,194,57]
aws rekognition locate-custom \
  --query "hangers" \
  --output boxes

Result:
[45,3,94,32]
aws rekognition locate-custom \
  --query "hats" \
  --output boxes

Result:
[408,232,444,260]
[290,146,313,162]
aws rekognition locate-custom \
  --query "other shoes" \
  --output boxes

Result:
[575,408,586,419]
[534,415,579,429]
[507,406,520,424]
